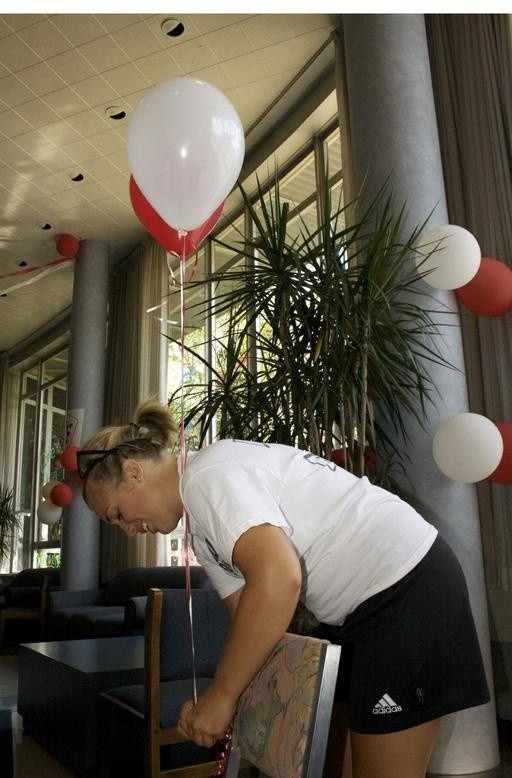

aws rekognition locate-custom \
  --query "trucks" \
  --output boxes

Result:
[455,256,512,318]
[127,75,246,238]
[36,445,91,524]
[488,422,511,485]
[433,413,503,483]
[129,173,225,260]
[415,224,481,291]
[56,232,80,258]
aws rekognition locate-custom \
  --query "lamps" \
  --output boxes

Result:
[0,568,59,655]
[225,628,342,777]
[100,588,258,778]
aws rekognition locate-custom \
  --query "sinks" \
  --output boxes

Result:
[51,567,214,641]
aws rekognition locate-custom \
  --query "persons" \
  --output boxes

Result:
[77,398,492,772]
